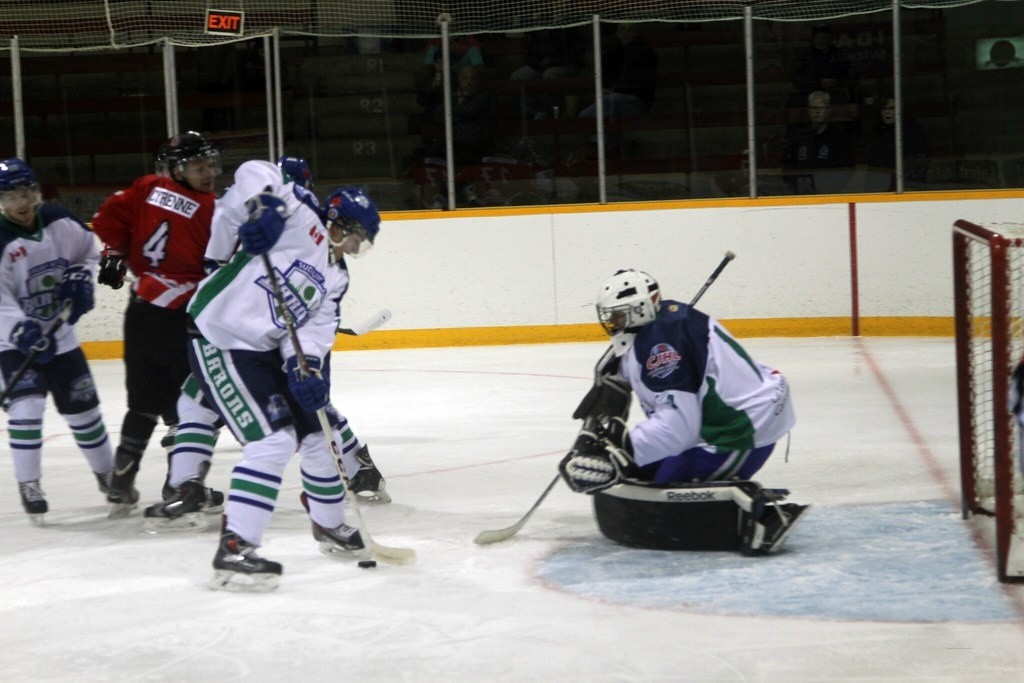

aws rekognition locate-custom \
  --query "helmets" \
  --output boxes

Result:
[276,155,314,190]
[325,188,380,244]
[596,270,661,357]
[0,158,35,192]
[156,131,216,169]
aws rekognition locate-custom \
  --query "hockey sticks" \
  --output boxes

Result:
[246,196,411,565]
[2,302,74,401]
[474,250,735,547]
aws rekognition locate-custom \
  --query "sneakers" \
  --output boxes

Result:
[18,481,49,527]
[161,471,225,516]
[755,503,812,555]
[340,444,392,502]
[161,422,177,447]
[107,447,140,518]
[96,470,140,503]
[211,514,283,592]
[300,491,370,558]
[143,461,210,534]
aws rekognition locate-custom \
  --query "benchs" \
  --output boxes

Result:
[0,0,1023,215]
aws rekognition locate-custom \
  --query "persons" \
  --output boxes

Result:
[92,130,225,517]
[402,0,660,208]
[1,158,139,526]
[186,160,380,592]
[780,24,933,193]
[561,269,812,554]
[144,153,391,537]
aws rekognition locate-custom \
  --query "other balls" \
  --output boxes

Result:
[357,561,376,568]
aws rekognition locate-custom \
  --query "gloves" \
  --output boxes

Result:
[9,320,58,365]
[53,263,94,325]
[238,192,288,255]
[286,354,329,412]
[204,260,229,274]
[97,245,127,290]
